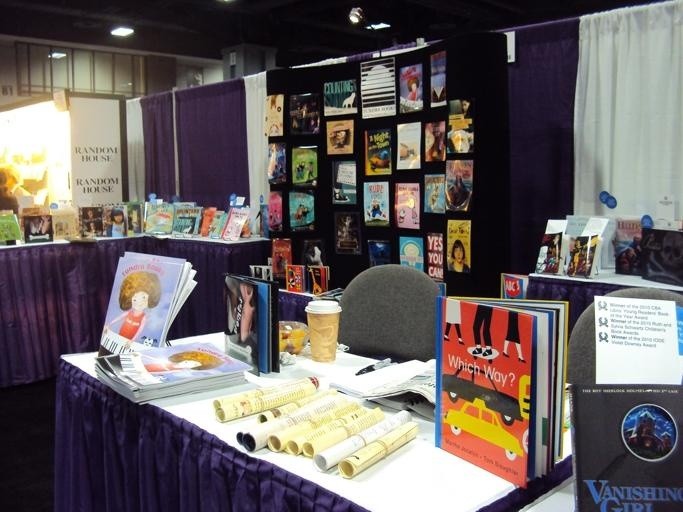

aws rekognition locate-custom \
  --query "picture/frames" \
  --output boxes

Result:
[23,215,53,242]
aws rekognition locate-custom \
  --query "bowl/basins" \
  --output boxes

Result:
[278,320,308,355]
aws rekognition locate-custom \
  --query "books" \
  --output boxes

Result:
[96,253,330,405]
[369,390,434,423]
[0,200,246,246]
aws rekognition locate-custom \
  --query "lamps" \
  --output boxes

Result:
[110,25,134,36]
[349,7,364,25]
[47,49,66,59]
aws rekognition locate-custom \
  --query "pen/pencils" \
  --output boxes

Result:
[355,357,392,376]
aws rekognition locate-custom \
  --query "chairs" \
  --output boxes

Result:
[565,286,683,391]
[337,263,443,363]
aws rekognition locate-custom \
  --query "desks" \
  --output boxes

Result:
[278,286,319,325]
[524,268,683,345]
[52,336,575,512]
[0,232,273,391]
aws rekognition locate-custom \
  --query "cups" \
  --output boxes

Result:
[305,299,341,361]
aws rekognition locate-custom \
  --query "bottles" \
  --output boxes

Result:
[1,208,22,241]
